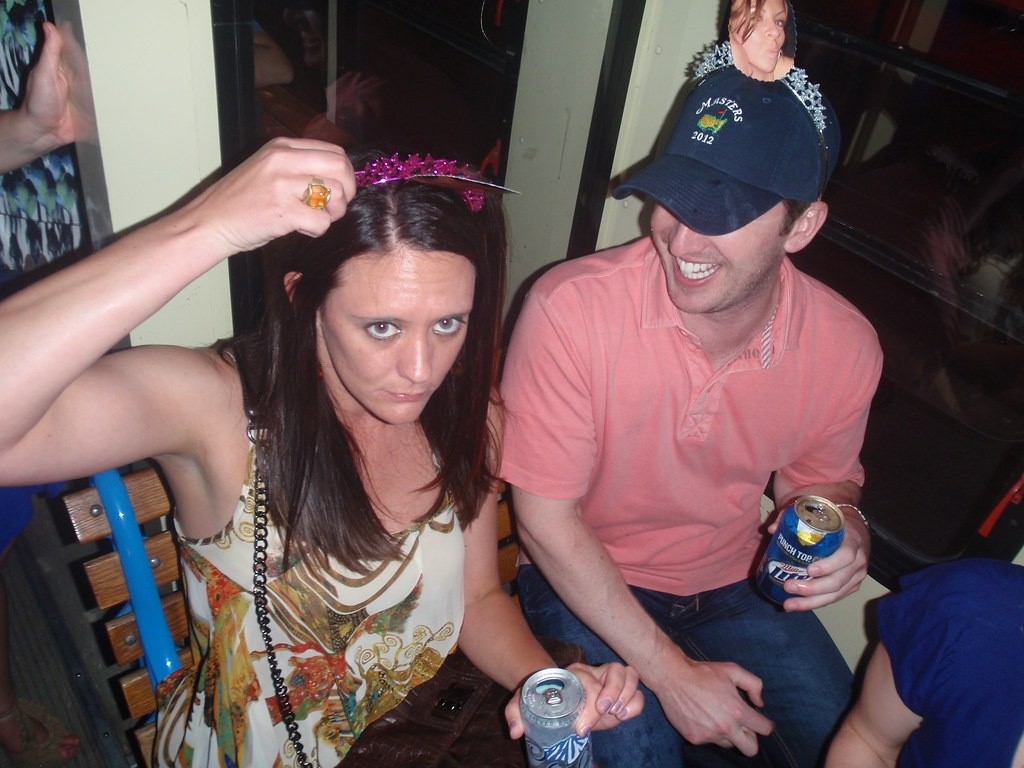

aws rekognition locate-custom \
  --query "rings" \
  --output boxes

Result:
[305,175,332,210]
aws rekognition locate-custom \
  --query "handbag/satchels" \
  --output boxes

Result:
[334,634,587,768]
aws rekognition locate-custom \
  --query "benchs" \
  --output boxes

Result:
[56,382,534,768]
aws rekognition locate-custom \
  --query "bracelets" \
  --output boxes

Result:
[835,503,870,528]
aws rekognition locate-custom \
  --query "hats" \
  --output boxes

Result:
[612,64,841,236]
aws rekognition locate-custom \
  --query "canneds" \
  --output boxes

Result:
[755,494,846,606]
[520,666,594,768]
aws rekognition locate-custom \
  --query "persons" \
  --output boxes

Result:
[484,65,885,767]
[826,558,1023,768]
[0,21,90,178]
[729,0,798,82]
[0,135,645,768]
